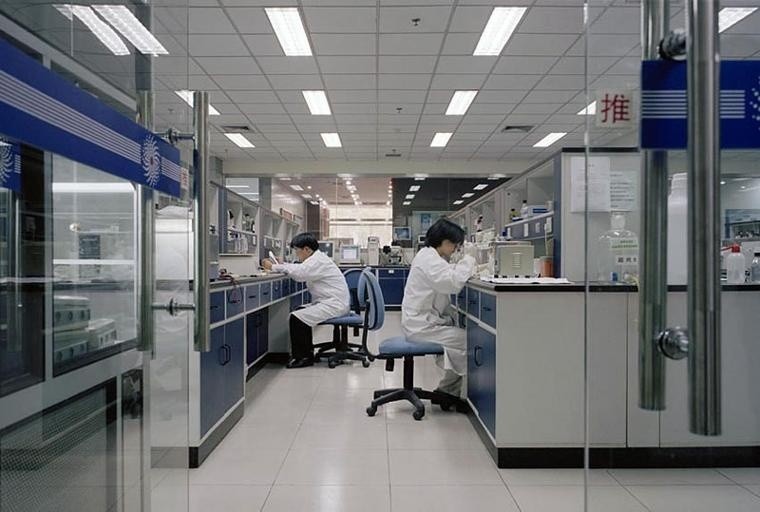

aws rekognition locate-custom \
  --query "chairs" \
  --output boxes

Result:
[314,265,453,421]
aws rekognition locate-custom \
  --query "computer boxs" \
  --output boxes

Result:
[368,236,379,265]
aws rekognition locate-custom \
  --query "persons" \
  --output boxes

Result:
[261,231,354,369]
[399,215,479,407]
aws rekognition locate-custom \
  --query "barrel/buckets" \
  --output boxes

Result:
[596,212,642,284]
[727,245,746,283]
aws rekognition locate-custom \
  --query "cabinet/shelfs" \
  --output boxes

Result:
[453,153,567,275]
[200,180,311,468]
[453,283,584,469]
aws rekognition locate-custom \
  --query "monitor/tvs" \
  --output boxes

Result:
[317,241,334,260]
[340,244,360,264]
[394,227,411,241]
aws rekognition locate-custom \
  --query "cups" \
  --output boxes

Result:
[538,254,555,277]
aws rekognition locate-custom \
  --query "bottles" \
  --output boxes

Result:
[508,205,517,220]
[521,198,529,221]
[725,244,745,285]
[750,251,760,286]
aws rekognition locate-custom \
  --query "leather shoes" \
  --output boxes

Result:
[288,358,313,368]
